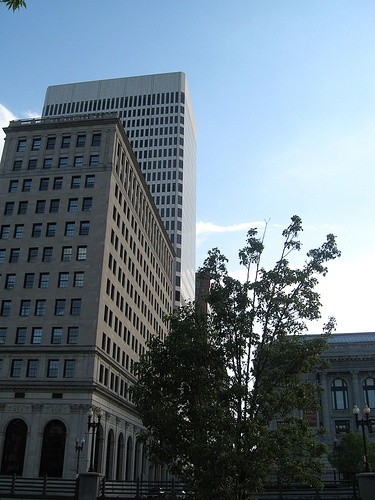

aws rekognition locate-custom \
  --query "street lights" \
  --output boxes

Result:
[86,407,103,473]
[75,437,85,474]
[352,404,374,473]
[333,437,342,479]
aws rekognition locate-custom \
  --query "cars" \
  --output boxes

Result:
[147,487,165,500]
[175,491,189,499]
